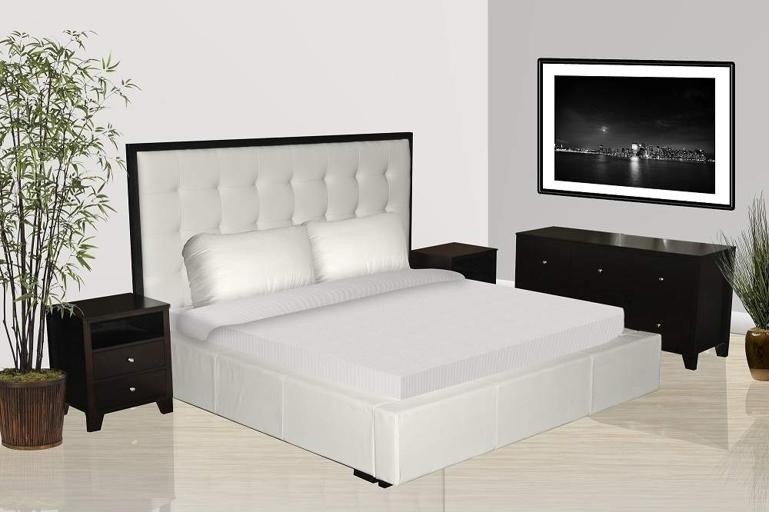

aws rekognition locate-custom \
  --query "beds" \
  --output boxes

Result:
[171,328,661,488]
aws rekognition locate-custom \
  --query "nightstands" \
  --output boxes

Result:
[46,291,173,432]
[410,240,497,282]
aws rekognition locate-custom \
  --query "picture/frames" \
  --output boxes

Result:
[536,57,736,211]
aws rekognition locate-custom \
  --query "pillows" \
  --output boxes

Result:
[182,225,316,309]
[303,211,409,283]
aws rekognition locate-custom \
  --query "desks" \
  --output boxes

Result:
[516,224,735,371]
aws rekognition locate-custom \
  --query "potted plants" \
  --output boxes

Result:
[0,24,142,451]
[714,188,769,381]
